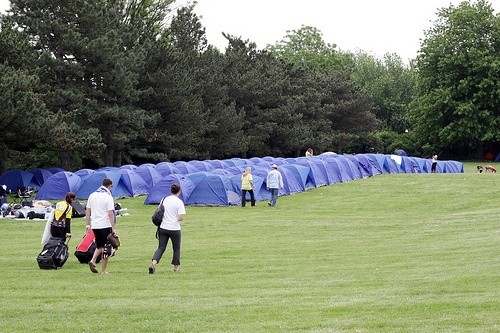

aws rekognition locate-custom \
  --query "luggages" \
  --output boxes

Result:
[36,234,71,270]
[74,229,103,263]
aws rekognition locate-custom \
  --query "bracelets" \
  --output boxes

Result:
[86,224,91,228]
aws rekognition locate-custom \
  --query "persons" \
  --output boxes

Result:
[86,178,116,274]
[305,148,313,158]
[148,183,186,274]
[266,164,284,207]
[477,165,497,174]
[430,153,437,173]
[241,167,256,207]
[41,192,76,244]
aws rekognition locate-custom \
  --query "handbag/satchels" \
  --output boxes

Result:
[50,219,66,237]
[103,233,120,259]
[152,195,169,226]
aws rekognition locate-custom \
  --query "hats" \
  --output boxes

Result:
[271,165,277,168]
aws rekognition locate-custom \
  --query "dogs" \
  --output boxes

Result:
[476,164,497,174]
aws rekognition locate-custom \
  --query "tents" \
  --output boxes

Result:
[0,152,466,205]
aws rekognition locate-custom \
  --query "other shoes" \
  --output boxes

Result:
[88,262,98,273]
[268,203,271,206]
[149,265,155,274]
